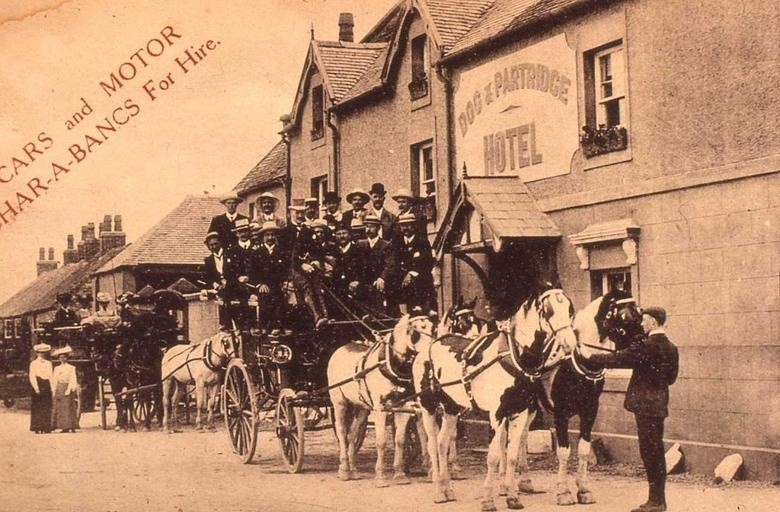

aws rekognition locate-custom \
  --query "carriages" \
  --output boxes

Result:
[0,316,95,414]
[219,273,647,510]
[83,311,237,432]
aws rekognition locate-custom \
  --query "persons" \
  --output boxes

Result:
[29,343,55,433]
[54,291,117,326]
[203,183,441,334]
[52,346,80,432]
[579,306,679,512]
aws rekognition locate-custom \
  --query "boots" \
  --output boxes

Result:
[631,487,666,512]
[303,294,335,331]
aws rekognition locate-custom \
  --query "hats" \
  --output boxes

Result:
[203,183,417,243]
[33,293,127,354]
[643,307,665,320]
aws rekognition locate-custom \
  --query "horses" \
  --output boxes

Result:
[414,295,481,481]
[160,331,236,436]
[326,309,435,488]
[411,280,578,512]
[498,281,649,505]
